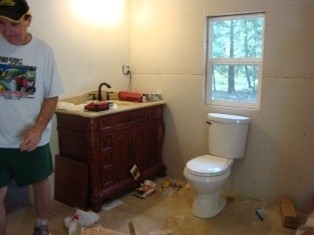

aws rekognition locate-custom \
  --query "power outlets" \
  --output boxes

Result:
[123,65,130,74]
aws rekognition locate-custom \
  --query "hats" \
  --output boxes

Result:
[0,0,30,23]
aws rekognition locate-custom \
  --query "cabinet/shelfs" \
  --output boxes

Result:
[54,100,165,212]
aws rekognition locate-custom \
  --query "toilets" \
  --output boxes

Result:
[182,112,251,218]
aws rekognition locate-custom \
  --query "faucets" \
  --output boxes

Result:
[98,82,112,101]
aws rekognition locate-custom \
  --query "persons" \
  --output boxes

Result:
[0,0,66,235]
